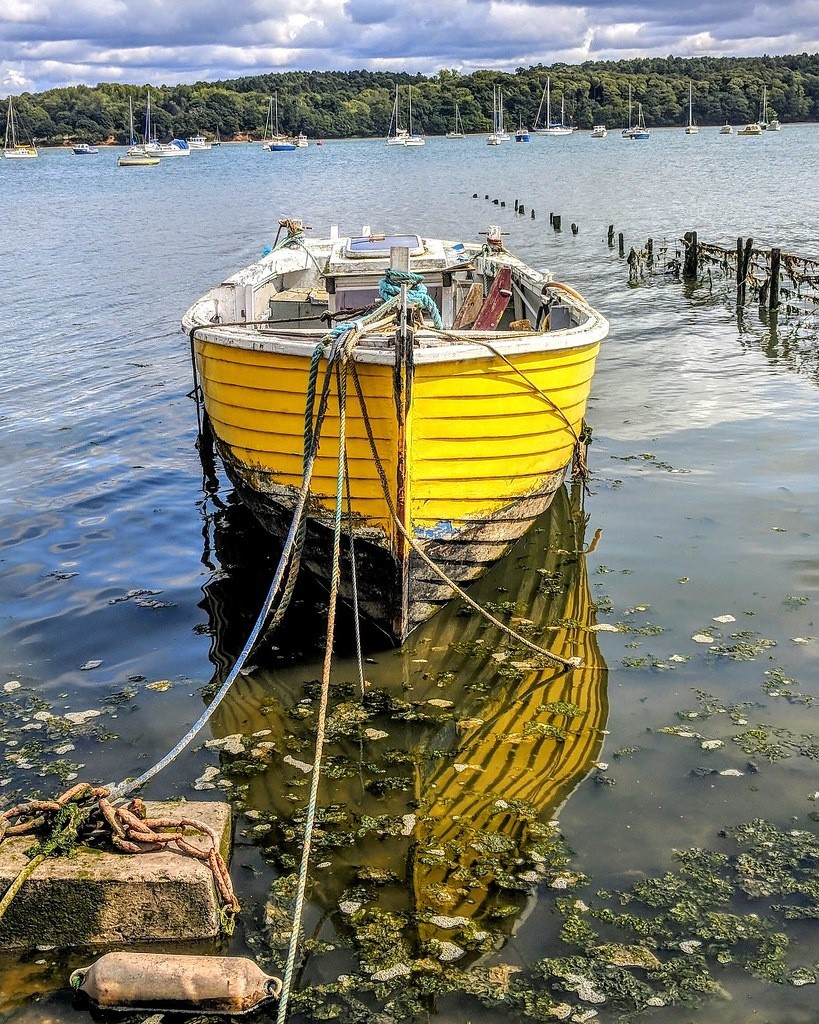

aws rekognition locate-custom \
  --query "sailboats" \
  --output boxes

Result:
[623,84,651,140]
[754,85,770,129]
[530,76,578,136]
[685,81,699,134]
[261,90,310,151]
[385,84,426,147]
[2,95,40,158]
[486,84,511,147]
[446,102,468,139]
[117,90,222,165]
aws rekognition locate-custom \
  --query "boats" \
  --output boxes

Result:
[182,226,609,638]
[183,485,611,989]
[766,120,781,132]
[737,125,763,136]
[719,121,734,134]
[71,142,100,154]
[515,114,530,143]
[592,125,607,138]
